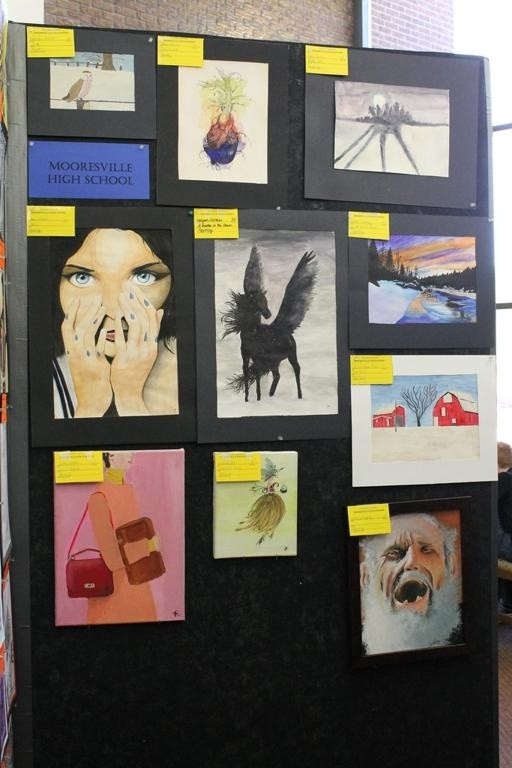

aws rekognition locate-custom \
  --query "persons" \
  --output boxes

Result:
[85,448,155,625]
[360,512,462,654]
[495,443,512,613]
[53,226,180,418]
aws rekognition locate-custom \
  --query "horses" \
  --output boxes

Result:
[219,288,302,401]
